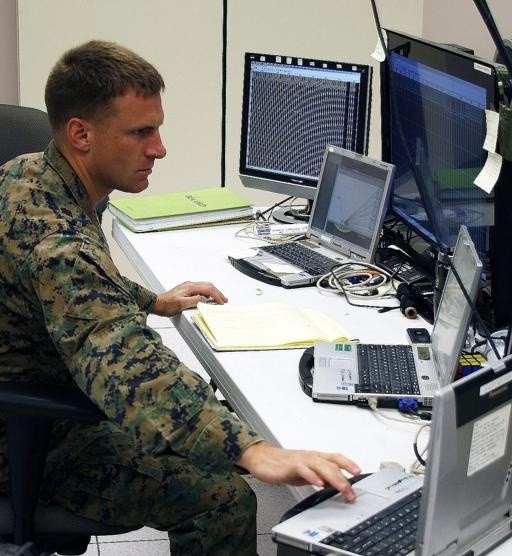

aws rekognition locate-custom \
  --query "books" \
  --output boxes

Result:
[190,298,360,353]
[106,186,256,233]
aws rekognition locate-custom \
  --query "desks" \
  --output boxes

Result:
[109,205,434,502]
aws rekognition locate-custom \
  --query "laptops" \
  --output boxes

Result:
[312,226,484,404]
[270,356,512,556]
[228,143,396,286]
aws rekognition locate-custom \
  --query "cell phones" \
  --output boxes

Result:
[406,327,430,343]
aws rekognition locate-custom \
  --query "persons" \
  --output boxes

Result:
[0,38,364,556]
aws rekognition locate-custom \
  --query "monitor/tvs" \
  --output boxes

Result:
[380,26,512,329]
[236,51,375,223]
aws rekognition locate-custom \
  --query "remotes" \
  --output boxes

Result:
[380,256,426,284]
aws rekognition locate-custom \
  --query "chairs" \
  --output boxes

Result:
[1,393,142,555]
[3,106,107,224]
